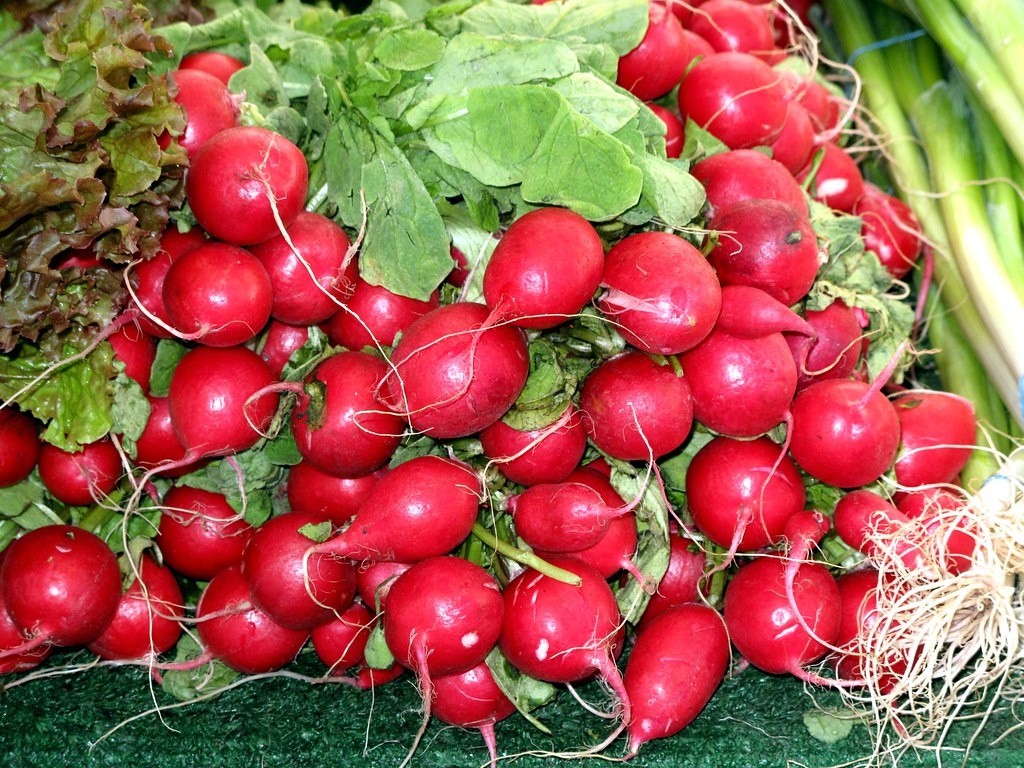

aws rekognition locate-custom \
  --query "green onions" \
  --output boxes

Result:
[805,0,1024,763]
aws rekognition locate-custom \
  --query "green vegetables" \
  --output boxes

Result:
[0,0,911,735]
